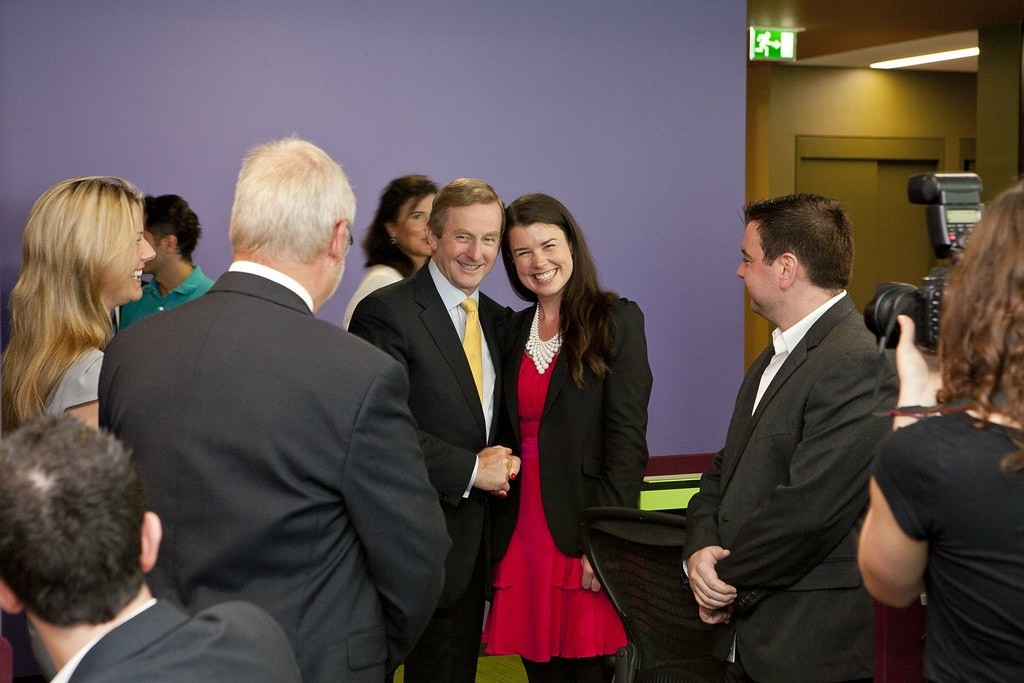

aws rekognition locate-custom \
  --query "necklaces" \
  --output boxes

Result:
[527,304,562,374]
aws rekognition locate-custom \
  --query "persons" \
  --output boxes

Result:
[858,178,1024,683]
[97,135,449,683]
[1,176,156,682]
[675,193,899,682]
[1,412,303,682]
[116,193,214,333]
[342,174,439,335]
[347,179,516,683]
[479,193,656,683]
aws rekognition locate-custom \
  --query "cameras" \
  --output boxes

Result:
[864,170,986,350]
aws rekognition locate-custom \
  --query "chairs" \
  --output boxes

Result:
[577,507,737,683]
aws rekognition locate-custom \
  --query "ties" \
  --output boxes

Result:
[459,297,483,403]
